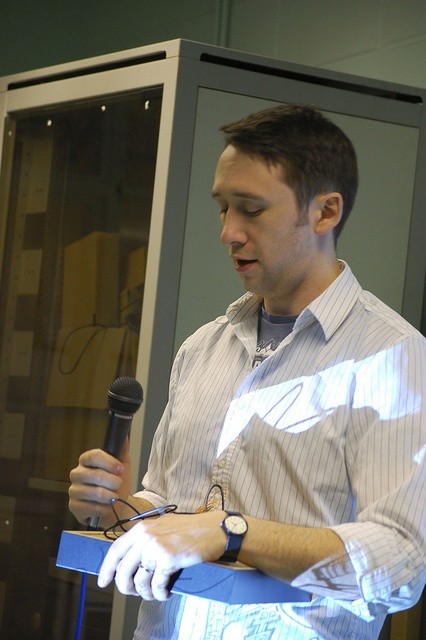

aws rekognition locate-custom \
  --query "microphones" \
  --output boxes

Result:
[88,377,143,530]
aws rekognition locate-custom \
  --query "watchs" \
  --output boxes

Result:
[217,509,248,567]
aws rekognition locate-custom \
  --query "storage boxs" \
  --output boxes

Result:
[41,324,139,481]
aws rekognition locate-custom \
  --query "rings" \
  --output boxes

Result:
[138,563,154,574]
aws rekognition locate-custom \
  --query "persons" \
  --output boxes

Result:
[67,103,426,639]
